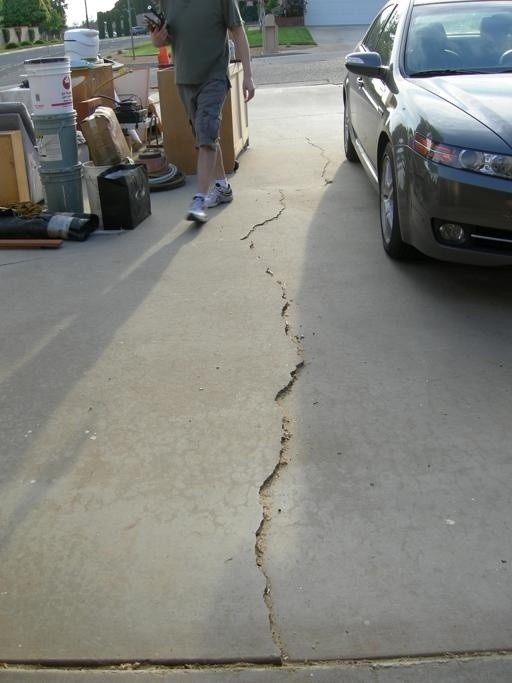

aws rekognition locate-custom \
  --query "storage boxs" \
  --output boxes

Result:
[79,107,132,166]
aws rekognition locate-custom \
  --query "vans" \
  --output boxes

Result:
[104,31,116,38]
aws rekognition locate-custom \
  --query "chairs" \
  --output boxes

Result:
[0,102,90,204]
[479,14,512,66]
[407,22,469,72]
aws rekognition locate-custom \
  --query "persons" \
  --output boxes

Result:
[149,0,256,222]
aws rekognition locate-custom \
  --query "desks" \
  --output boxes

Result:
[0,86,121,113]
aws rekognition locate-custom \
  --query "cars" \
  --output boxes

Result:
[341,0,510,272]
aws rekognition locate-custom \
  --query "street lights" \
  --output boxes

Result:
[103,21,108,32]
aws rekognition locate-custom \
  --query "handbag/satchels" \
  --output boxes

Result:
[96,162,153,232]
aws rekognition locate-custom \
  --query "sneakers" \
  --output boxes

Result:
[184,194,208,223]
[204,182,234,208]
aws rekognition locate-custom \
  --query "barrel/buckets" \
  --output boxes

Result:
[30,112,79,169]
[23,55,75,114]
[36,162,82,214]
[82,161,125,217]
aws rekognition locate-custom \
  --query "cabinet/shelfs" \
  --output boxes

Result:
[157,61,249,174]
[71,62,116,130]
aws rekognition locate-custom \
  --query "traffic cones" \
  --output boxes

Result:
[155,45,170,68]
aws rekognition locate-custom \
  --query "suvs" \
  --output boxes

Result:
[131,25,148,35]
